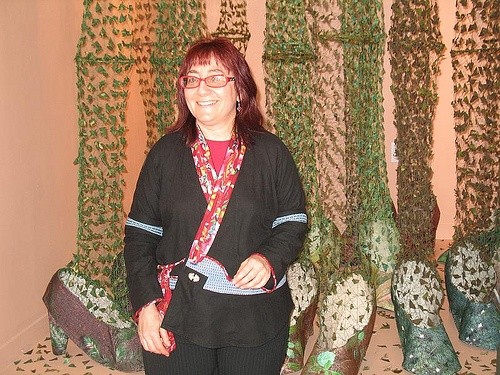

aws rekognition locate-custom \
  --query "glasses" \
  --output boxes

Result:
[178,75,237,88]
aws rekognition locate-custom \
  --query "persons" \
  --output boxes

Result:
[118,33,314,375]
